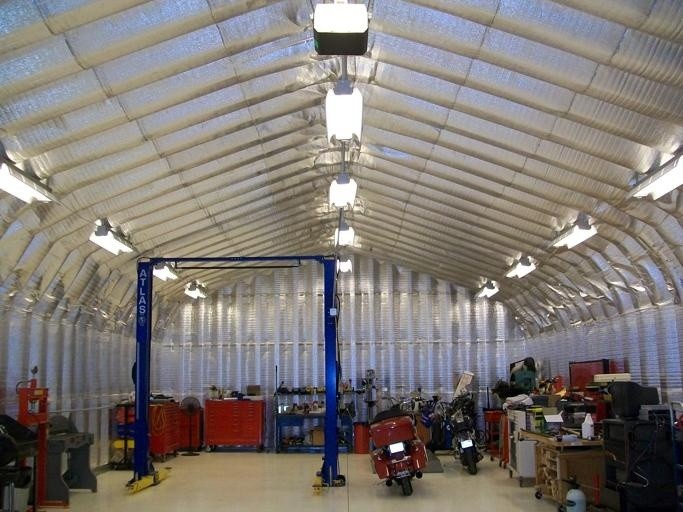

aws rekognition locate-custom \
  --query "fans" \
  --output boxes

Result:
[180,396,201,456]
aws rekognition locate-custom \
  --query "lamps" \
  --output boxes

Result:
[474,154,683,299]
[0,156,208,299]
[310,0,372,273]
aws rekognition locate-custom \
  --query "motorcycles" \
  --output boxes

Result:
[368,387,488,496]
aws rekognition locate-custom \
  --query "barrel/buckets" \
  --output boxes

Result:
[581,412,594,441]
[565,488,587,512]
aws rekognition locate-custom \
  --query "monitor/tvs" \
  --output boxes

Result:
[604,380,660,418]
[247,385,260,396]
[602,420,673,470]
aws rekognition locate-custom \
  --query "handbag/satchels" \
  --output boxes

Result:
[421,412,444,427]
